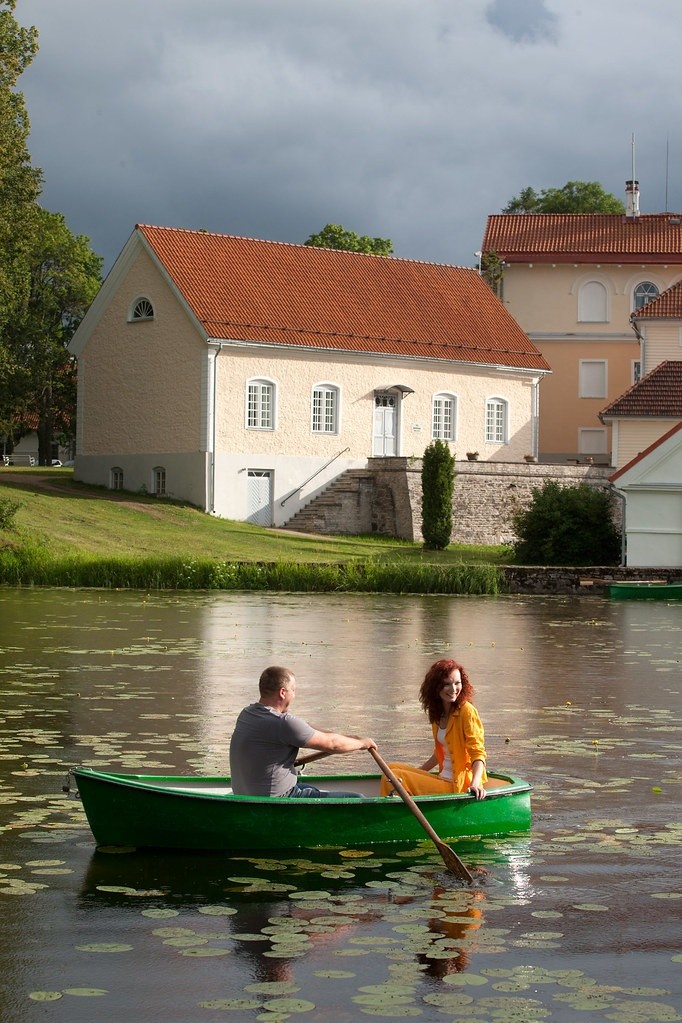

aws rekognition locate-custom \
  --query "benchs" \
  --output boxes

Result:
[2,454,36,467]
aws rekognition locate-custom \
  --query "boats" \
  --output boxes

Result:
[82,838,530,905]
[74,763,532,851]
[604,581,682,603]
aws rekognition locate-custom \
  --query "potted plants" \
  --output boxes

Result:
[466,450,480,461]
[524,454,538,462]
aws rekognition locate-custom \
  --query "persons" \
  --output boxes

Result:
[380,659,489,800]
[229,666,378,798]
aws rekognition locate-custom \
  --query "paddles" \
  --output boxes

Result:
[296,751,329,767]
[370,745,473,884]
